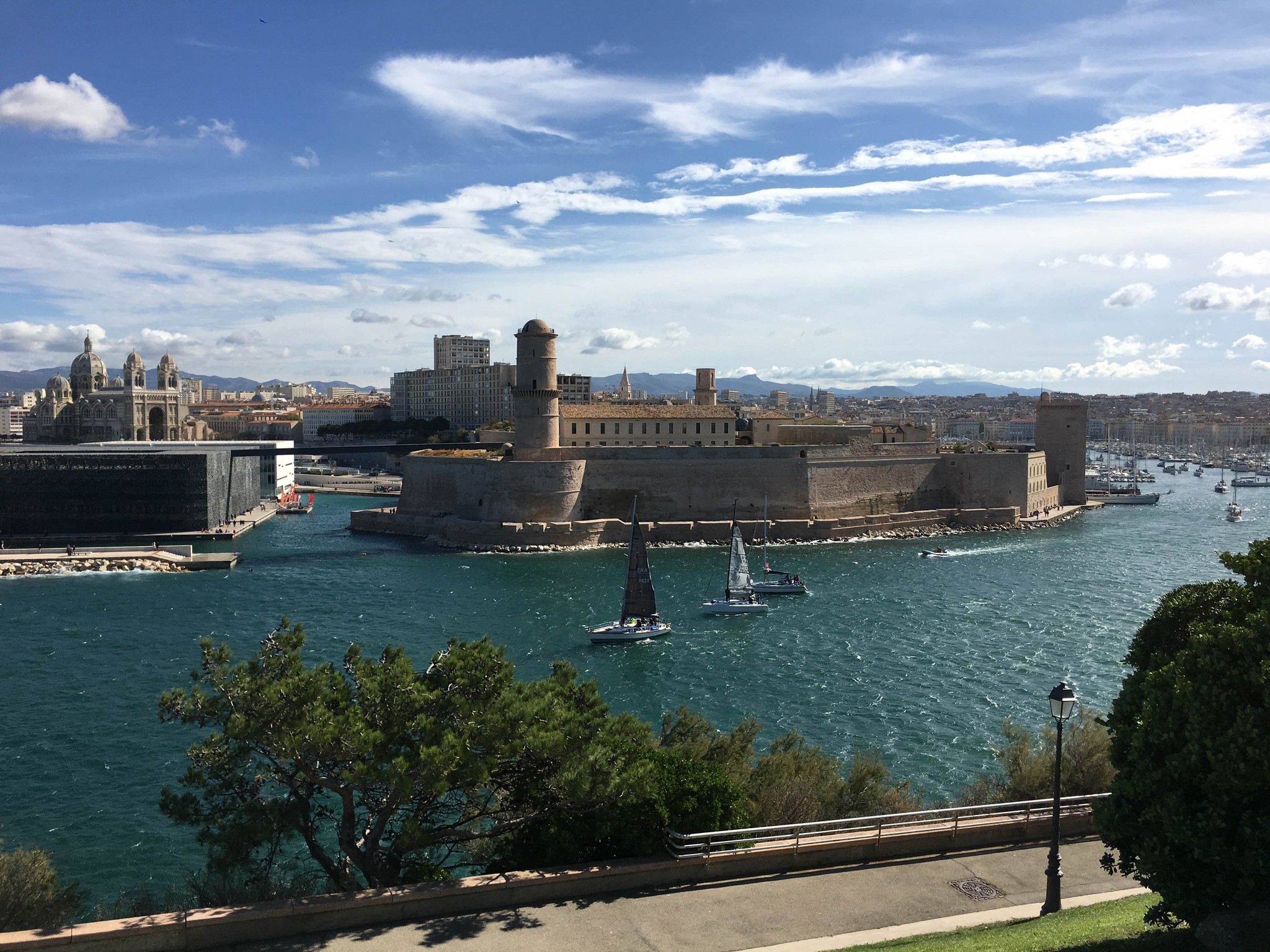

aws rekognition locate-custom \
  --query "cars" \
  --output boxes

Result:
[369,471,379,476]
[380,468,388,471]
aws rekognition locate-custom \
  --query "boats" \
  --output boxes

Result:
[921,546,948,557]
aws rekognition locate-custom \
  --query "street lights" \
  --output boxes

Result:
[376,464,379,480]
[1037,680,1079,920]
[351,458,354,468]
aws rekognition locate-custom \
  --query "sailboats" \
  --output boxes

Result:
[585,493,673,642]
[747,492,811,593]
[1084,416,1270,522]
[701,498,769,614]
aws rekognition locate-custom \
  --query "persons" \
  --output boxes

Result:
[724,588,759,604]
[785,574,799,585]
[304,480,400,493]
[0,540,76,556]
[635,617,659,629]
[201,503,266,533]
[937,547,942,553]
[151,542,160,551]
[389,508,395,514]
